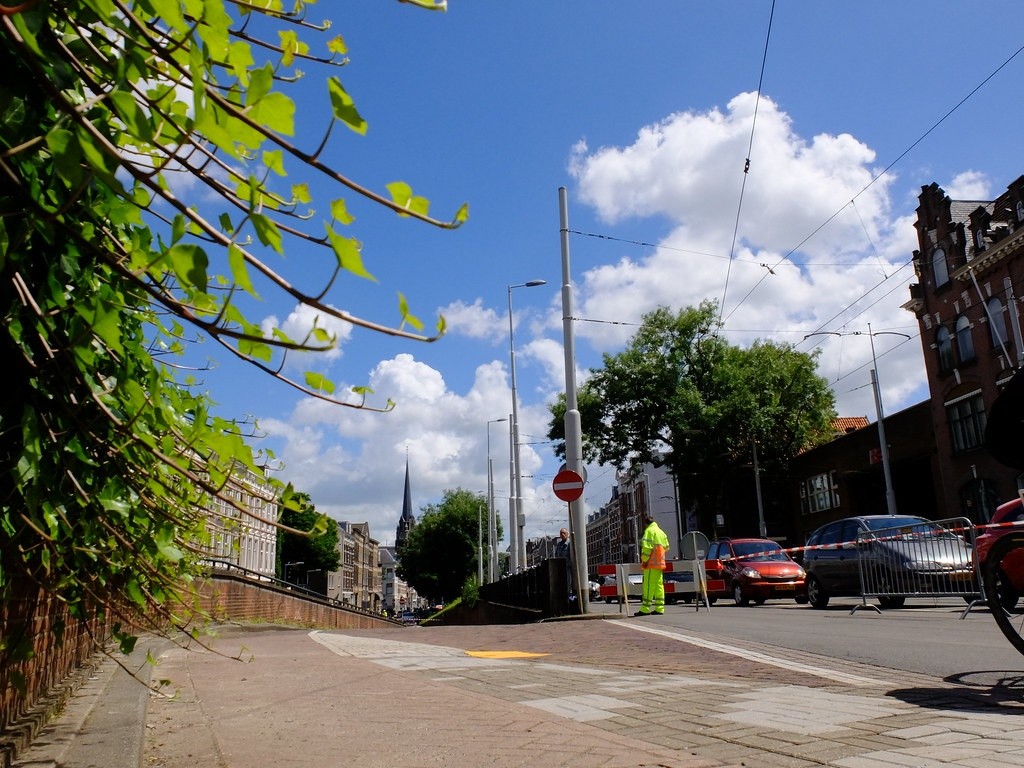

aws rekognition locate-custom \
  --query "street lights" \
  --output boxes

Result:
[508,279,548,572]
[485,417,508,585]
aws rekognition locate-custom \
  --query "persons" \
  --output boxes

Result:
[555,528,578,602]
[634,516,670,616]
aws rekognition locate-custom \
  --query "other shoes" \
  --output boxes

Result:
[651,610,663,615]
[634,610,650,616]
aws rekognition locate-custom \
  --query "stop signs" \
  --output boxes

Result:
[553,470,584,503]
[400,599,405,605]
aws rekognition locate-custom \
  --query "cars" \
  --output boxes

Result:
[395,609,441,625]
[803,514,987,610]
[585,569,701,604]
[705,535,809,607]
[973,498,1024,611]
